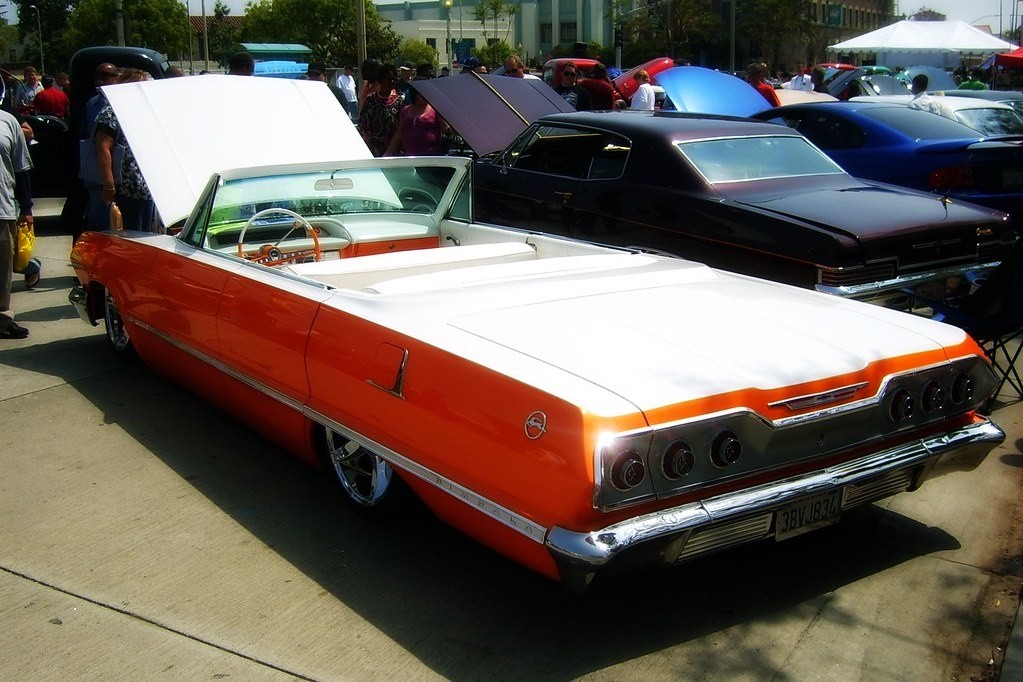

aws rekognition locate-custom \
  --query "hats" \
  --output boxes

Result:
[745,63,762,78]
[459,56,480,68]
[344,65,355,72]
[41,75,53,84]
[400,61,413,71]
[307,62,325,72]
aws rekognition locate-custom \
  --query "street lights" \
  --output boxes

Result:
[443,0,454,73]
[30,5,45,76]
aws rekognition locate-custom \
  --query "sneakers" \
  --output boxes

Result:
[0,321,29,338]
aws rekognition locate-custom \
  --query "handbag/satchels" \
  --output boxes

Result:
[106,200,124,232]
[78,106,124,185]
[14,221,35,272]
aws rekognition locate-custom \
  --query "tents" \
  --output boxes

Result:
[823,20,1023,92]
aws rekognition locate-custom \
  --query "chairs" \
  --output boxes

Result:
[587,151,629,179]
[899,238,1023,415]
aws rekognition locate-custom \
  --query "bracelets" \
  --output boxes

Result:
[104,188,115,191]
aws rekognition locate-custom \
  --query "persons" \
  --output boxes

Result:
[229,52,255,77]
[780,61,1023,98]
[580,64,615,110]
[552,61,584,112]
[0,63,211,338]
[504,55,541,80]
[629,70,655,111]
[336,64,358,119]
[357,56,489,158]
[594,55,602,62]
[741,62,781,109]
[907,74,940,116]
[305,61,349,115]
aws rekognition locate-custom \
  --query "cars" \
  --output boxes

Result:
[62,47,1023,587]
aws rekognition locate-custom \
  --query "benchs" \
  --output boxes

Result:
[284,242,538,291]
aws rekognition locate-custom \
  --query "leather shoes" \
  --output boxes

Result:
[25,270,39,288]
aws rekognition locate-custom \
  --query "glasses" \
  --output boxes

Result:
[564,70,577,78]
[505,65,520,74]
[308,71,324,77]
